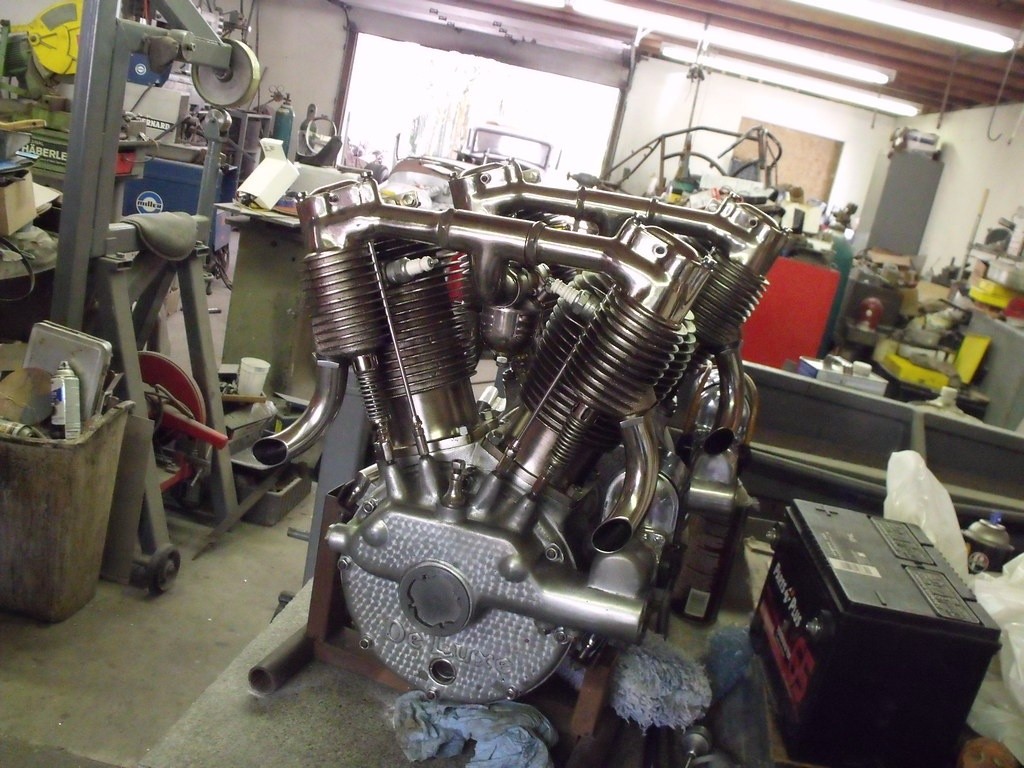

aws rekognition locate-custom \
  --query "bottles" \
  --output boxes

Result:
[49,359,81,440]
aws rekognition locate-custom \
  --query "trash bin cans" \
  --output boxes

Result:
[0,396,138,624]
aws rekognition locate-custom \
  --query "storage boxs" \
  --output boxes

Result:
[228,401,279,452]
[122,156,237,213]
[748,498,1004,768]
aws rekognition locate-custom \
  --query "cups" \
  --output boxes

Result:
[238,357,271,396]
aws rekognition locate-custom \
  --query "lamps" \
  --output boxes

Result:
[659,39,923,118]
[799,0,1024,54]
[575,1,896,90]
